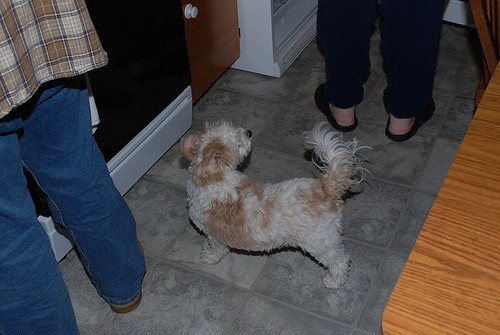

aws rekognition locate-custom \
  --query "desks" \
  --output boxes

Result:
[381,61,500,335]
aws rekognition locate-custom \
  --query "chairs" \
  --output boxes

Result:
[468,0,500,108]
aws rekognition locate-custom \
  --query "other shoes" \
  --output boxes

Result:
[110,239,145,314]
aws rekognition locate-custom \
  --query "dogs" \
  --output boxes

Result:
[179,118,374,289]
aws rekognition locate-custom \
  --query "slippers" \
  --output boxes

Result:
[314,82,358,133]
[385,96,435,142]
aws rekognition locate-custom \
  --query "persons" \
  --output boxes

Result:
[315,0,446,142]
[0,0,147,335]
